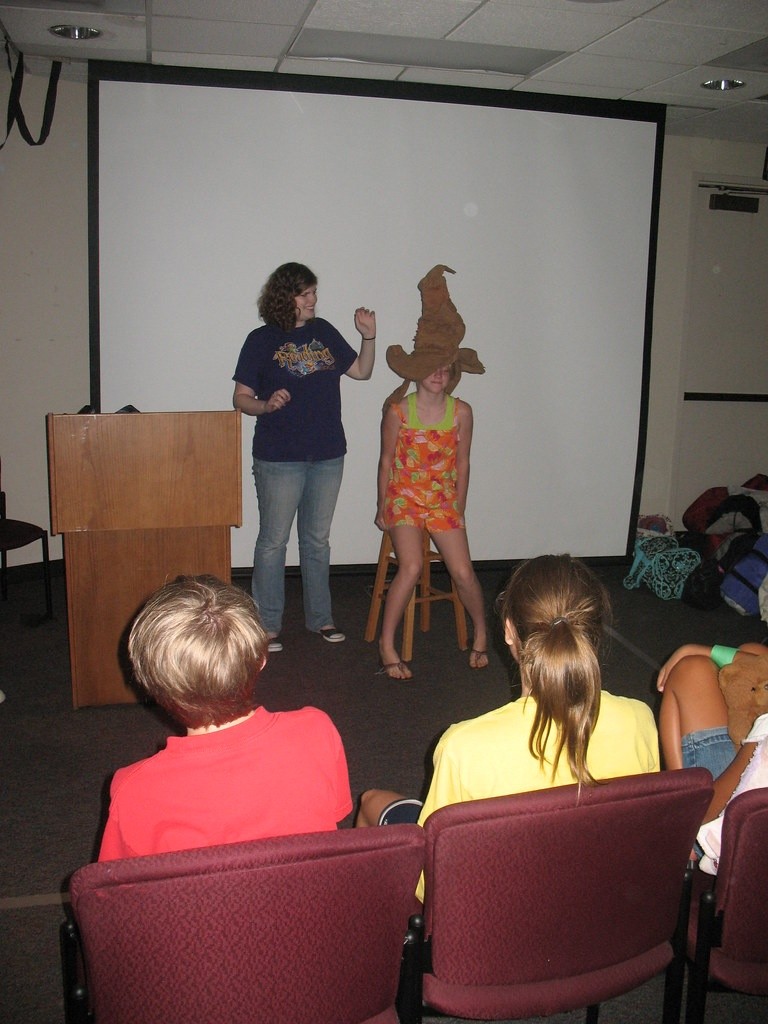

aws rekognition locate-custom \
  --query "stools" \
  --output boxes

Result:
[365,518,468,651]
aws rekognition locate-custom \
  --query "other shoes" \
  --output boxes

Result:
[267,638,284,651]
[318,627,344,643]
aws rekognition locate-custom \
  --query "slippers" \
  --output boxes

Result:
[379,649,413,683]
[468,646,490,674]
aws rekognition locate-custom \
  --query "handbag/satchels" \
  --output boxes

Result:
[624,474,768,623]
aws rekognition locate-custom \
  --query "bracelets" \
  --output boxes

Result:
[711,645,740,668]
[363,337,376,340]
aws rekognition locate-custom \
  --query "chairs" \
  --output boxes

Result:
[0,492,54,631]
[64,759,768,1024]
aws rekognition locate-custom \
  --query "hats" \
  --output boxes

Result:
[383,264,485,418]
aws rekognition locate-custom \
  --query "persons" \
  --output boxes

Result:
[659,642,768,873]
[355,553,659,904]
[97,575,352,863]
[375,364,489,682]
[233,262,376,652]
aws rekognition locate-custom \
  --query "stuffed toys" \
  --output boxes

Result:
[719,654,768,744]
